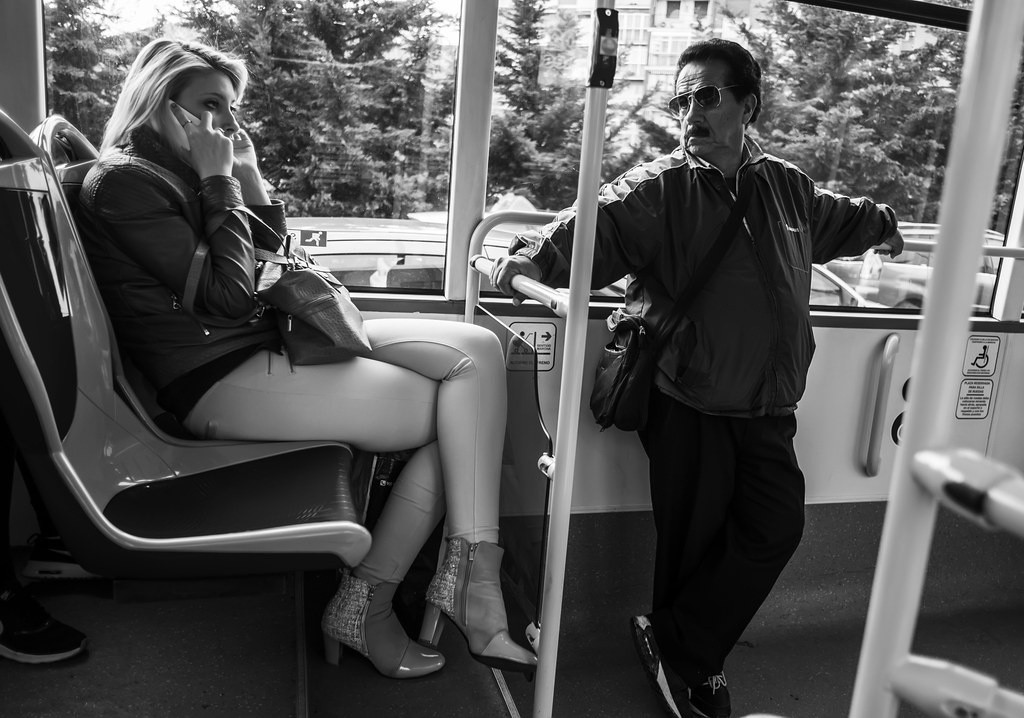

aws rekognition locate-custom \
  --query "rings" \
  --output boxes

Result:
[216,128,224,134]
[182,120,191,128]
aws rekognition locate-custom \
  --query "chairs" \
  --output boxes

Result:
[0,109,372,718]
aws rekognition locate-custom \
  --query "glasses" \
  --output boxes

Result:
[670,83,739,116]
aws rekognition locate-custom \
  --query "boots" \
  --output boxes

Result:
[321,573,445,678]
[419,536,538,674]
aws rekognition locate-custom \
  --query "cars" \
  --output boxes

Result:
[255,212,1007,312]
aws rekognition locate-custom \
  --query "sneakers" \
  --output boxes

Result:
[686,661,731,718]
[0,577,87,664]
[22,532,106,579]
[630,612,692,718]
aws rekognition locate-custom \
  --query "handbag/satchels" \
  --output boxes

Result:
[182,206,373,367]
[589,308,662,431]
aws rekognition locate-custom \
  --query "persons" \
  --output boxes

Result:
[78,37,541,679]
[490,37,903,718]
[0,334,117,664]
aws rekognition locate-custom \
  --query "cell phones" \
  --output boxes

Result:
[151,97,203,152]
